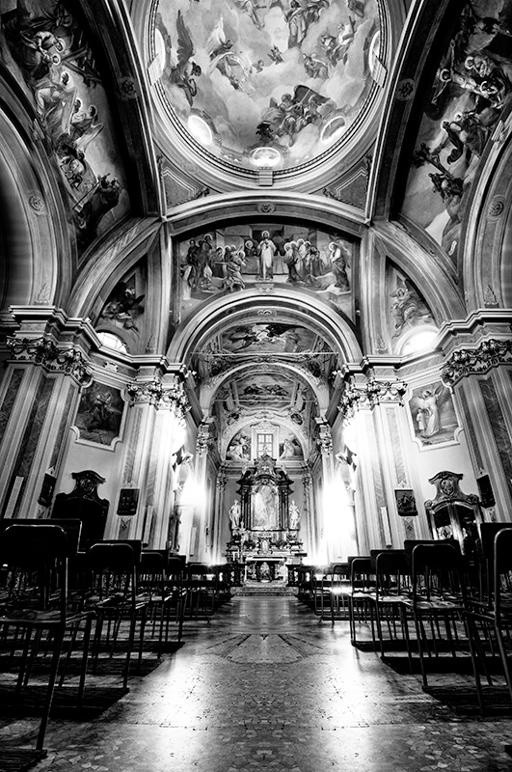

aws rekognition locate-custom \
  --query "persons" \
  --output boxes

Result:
[14,3,124,243]
[421,385,445,436]
[158,0,369,151]
[417,407,428,429]
[228,499,242,530]
[182,230,351,295]
[411,7,510,205]
[288,499,300,528]
[280,438,299,458]
[226,435,252,462]
